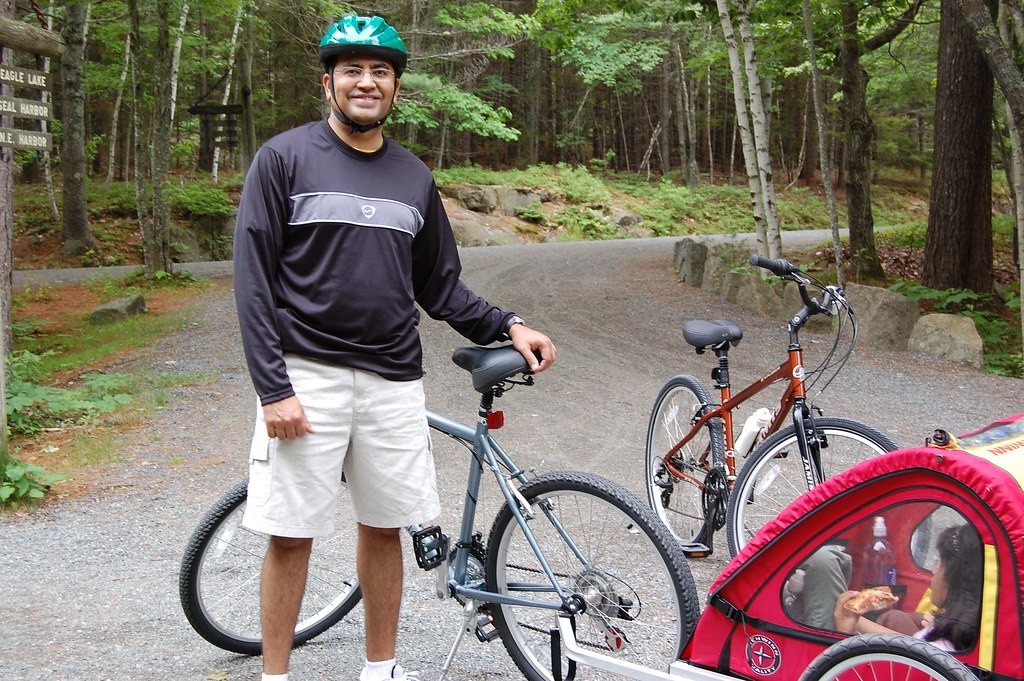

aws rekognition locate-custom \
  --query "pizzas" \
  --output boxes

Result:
[844,585,898,614]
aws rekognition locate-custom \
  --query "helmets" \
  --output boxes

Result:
[318,15,407,79]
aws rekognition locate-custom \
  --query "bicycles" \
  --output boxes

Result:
[645,253,903,563]
[177,343,701,681]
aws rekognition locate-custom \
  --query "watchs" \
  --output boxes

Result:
[502,315,526,341]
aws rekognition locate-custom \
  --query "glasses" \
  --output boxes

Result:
[331,66,396,80]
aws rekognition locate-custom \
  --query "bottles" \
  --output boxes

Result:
[782,568,806,624]
[858,515,900,620]
[733,408,772,461]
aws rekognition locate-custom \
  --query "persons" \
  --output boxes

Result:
[234,13,556,681]
[803,524,985,653]
